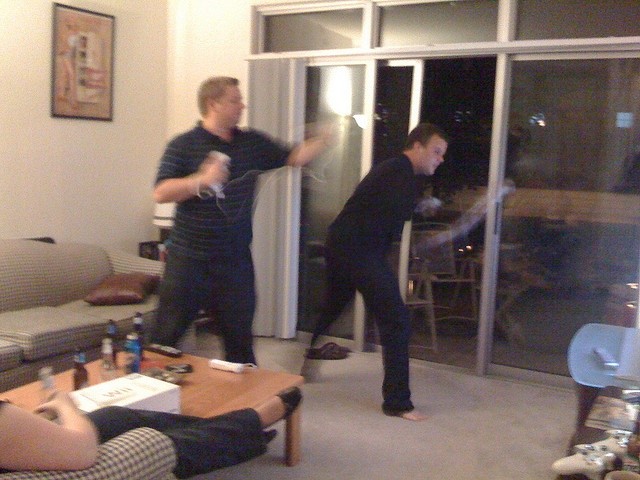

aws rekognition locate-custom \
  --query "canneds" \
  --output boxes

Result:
[126,334,141,376]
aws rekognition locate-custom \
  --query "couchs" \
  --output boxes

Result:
[1,238,198,395]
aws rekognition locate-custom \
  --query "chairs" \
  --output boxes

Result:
[409,219,480,354]
[375,233,440,354]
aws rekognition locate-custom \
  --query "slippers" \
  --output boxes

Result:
[310,342,349,352]
[307,348,346,360]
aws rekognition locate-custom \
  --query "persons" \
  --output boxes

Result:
[308,122,456,421]
[153,77,340,363]
[0,383,302,479]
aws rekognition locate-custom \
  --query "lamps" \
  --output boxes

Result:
[151,197,178,248]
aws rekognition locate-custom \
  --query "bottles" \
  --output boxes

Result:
[72,347,87,390]
[123,334,141,374]
[132,313,144,361]
[37,366,56,399]
[100,338,114,371]
[106,319,115,338]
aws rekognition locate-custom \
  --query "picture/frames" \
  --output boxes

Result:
[50,2,116,122]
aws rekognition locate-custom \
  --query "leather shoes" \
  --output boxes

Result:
[262,430,277,445]
[253,386,302,429]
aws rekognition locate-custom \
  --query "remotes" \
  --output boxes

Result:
[592,345,620,370]
[143,341,183,358]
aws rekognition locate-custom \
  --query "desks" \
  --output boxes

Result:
[1,345,305,468]
[554,385,640,479]
[565,321,638,395]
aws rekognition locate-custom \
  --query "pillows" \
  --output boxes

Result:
[83,269,161,306]
